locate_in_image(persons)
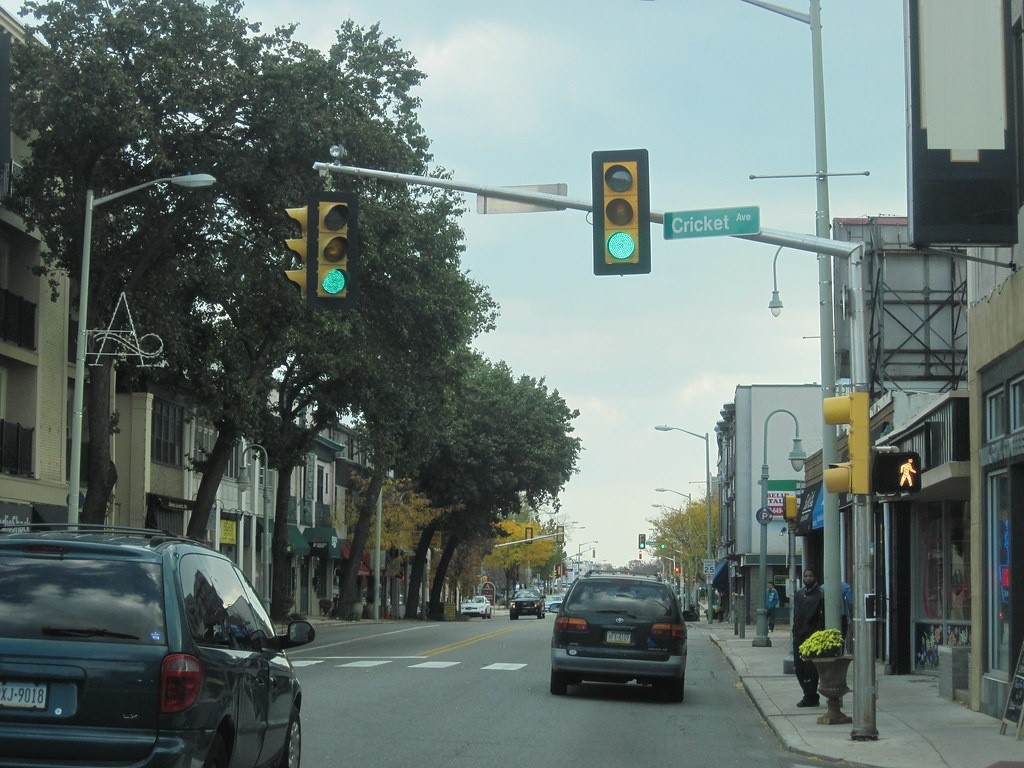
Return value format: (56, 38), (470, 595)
(619, 585), (631, 598)
(765, 581), (780, 633)
(792, 568), (825, 707)
(819, 581), (853, 655)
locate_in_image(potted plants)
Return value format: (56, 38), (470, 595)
(798, 628), (852, 725)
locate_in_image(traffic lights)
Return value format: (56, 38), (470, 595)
(285, 204), (308, 291)
(639, 534), (645, 549)
(316, 200), (353, 299)
(602, 160), (641, 265)
(821, 391), (870, 495)
(876, 450), (921, 494)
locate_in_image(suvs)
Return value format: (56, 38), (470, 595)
(0, 522), (317, 768)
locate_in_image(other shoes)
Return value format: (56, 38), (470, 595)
(797, 695), (819, 707)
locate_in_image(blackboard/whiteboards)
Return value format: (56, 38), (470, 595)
(1002, 641), (1024, 728)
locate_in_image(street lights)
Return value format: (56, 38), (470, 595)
(750, 408), (807, 648)
(235, 443), (271, 620)
(654, 425), (713, 625)
(65, 170), (220, 534)
(655, 487), (691, 613)
(577, 540), (598, 575)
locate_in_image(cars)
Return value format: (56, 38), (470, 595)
(508, 588), (546, 620)
(548, 568), (699, 703)
(460, 595), (492, 620)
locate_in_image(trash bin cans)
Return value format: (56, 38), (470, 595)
(690, 603), (700, 622)
